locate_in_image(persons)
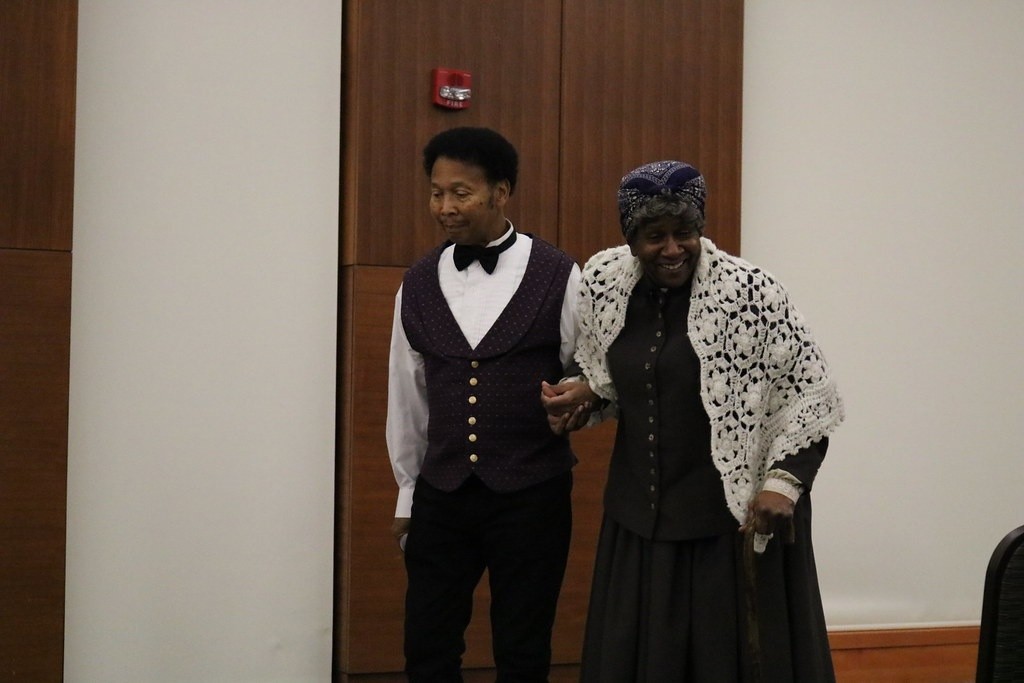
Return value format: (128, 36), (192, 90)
(541, 161), (846, 682)
(385, 127), (592, 683)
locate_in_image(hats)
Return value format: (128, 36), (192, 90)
(618, 160), (708, 233)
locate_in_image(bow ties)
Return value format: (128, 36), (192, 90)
(453, 226), (517, 275)
